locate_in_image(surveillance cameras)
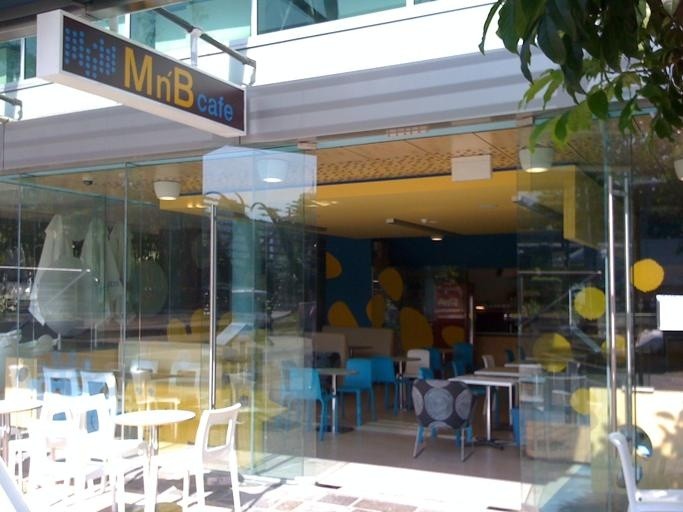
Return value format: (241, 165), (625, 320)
(83, 174), (94, 185)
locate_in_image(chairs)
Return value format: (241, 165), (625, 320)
(0, 388), (243, 511)
(40, 357), (205, 443)
(281, 324), (548, 464)
(167, 305), (238, 338)
(606, 429), (683, 511)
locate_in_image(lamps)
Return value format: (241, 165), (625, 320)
(517, 147), (555, 173)
(153, 179), (184, 202)
(260, 159), (288, 183)
